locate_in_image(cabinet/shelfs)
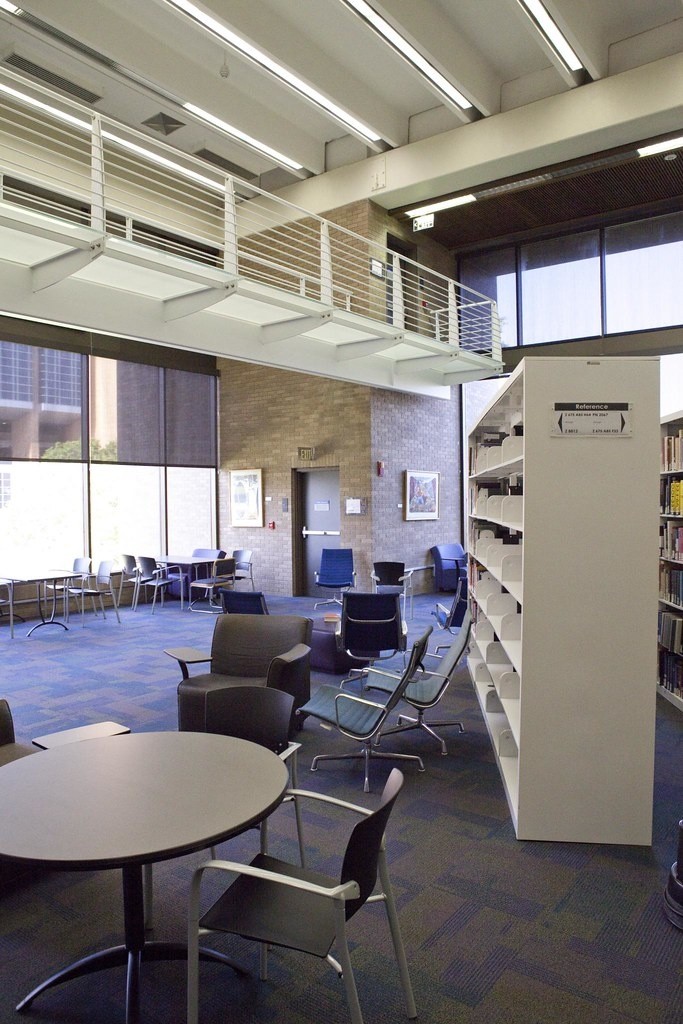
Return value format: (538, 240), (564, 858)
(467, 352), (660, 847)
(659, 409), (683, 713)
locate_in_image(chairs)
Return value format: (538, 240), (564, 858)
(202, 687), (304, 868)
(298, 627), (434, 793)
(0, 699), (44, 767)
(357, 606), (476, 755)
(120, 548), (266, 615)
(431, 577), (468, 663)
(183, 767), (419, 1024)
(369, 560), (414, 620)
(314, 548), (357, 610)
(163, 615), (313, 743)
(44, 558), (119, 626)
(336, 595), (409, 690)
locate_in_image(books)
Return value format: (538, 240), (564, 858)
(659, 430), (683, 697)
(324, 613), (340, 622)
(469, 432), (508, 623)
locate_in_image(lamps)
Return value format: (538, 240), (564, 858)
(219, 51), (230, 79)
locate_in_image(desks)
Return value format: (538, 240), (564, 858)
(155, 558), (236, 605)
(32, 721), (131, 750)
(310, 616), (367, 674)
(0, 732), (290, 1024)
(0, 572), (82, 636)
(163, 645), (212, 680)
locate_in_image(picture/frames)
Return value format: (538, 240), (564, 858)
(228, 469), (266, 528)
(404, 470), (439, 523)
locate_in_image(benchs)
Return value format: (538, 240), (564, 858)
(166, 549), (226, 598)
(431, 541), (469, 593)
